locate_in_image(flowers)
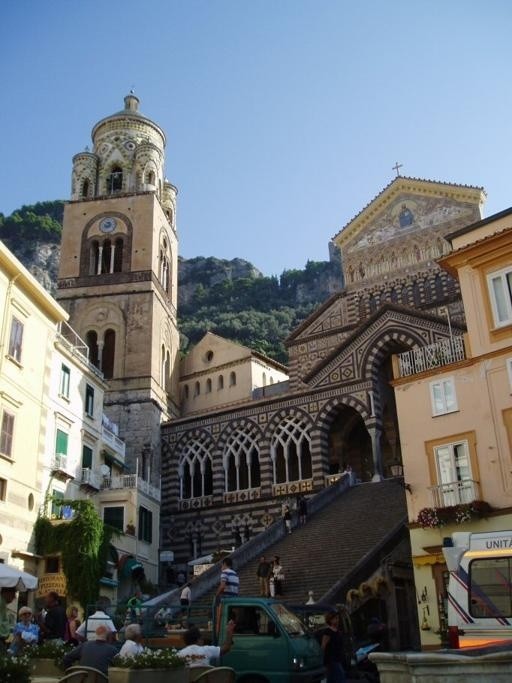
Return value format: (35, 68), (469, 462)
(416, 499), (492, 529)
(1, 638), (76, 682)
(111, 644), (189, 669)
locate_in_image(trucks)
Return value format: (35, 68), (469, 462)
(283, 598), (355, 680)
(364, 528), (512, 683)
(81, 594), (326, 683)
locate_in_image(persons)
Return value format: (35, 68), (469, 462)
(152, 601), (173, 630)
(317, 609), (349, 682)
(255, 555), (271, 597)
(64, 600), (82, 643)
(61, 623), (118, 678)
(8, 605), (43, 665)
(125, 589), (145, 624)
(73, 598), (118, 643)
(179, 580), (193, 617)
(119, 621), (147, 658)
(215, 557), (241, 596)
(36, 590), (67, 644)
(295, 492), (308, 525)
(170, 617), (237, 665)
(284, 503), (293, 534)
(0, 586), (18, 650)
(271, 555), (285, 595)
(267, 573), (276, 597)
